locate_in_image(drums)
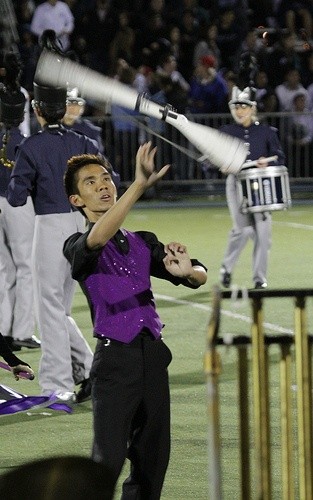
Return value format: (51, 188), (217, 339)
(235, 163), (295, 215)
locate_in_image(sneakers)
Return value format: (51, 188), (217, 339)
(12, 335), (41, 348)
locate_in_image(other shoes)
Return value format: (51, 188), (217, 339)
(253, 282), (264, 289)
(77, 378), (92, 403)
(220, 271), (231, 291)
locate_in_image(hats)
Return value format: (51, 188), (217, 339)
(33, 79), (67, 103)
(228, 86), (257, 108)
(196, 55), (214, 67)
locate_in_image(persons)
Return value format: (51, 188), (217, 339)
(62, 139), (207, 500)
(0, 76), (103, 404)
(12, 0), (313, 200)
(218, 85), (291, 286)
